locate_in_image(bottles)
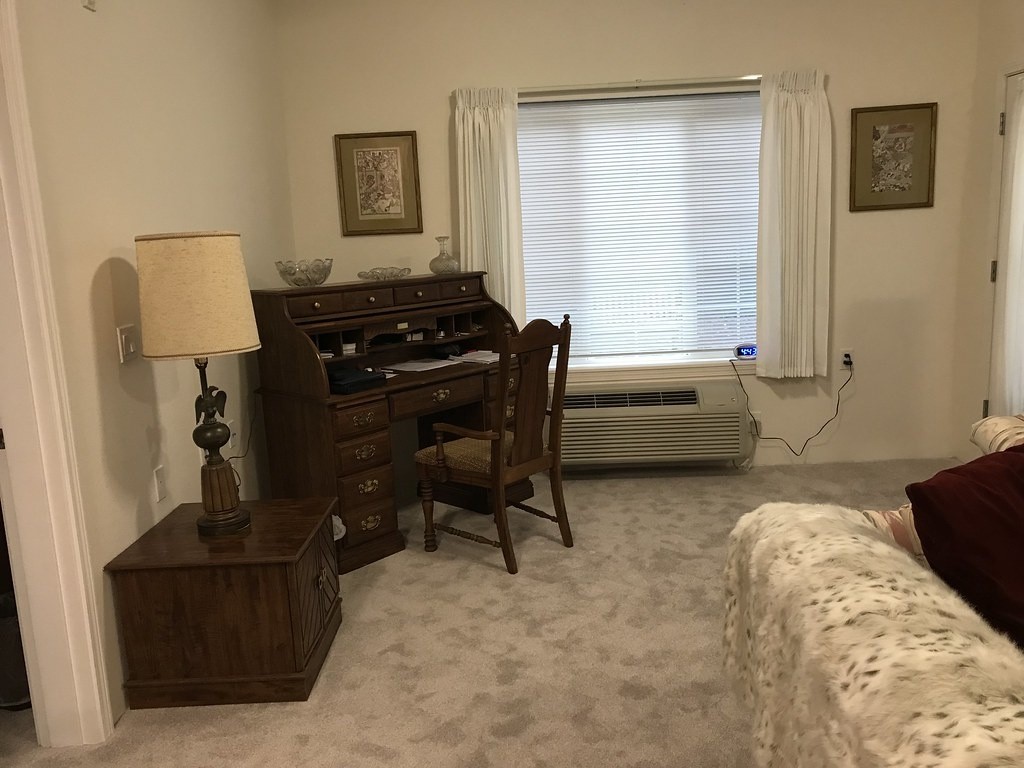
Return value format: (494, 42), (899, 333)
(429, 237), (458, 274)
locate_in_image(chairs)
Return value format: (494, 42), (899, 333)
(411, 313), (574, 574)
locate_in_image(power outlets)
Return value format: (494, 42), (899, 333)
(227, 420), (240, 449)
(747, 412), (763, 433)
(840, 348), (855, 370)
(199, 447), (209, 467)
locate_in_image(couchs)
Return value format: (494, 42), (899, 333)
(721, 415), (1024, 768)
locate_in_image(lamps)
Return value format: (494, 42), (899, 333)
(135, 229), (262, 536)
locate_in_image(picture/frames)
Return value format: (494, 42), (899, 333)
(334, 130), (423, 237)
(849, 102), (939, 211)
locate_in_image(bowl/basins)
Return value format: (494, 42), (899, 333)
(275, 258), (333, 285)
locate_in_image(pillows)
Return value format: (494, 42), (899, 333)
(904, 443), (1024, 654)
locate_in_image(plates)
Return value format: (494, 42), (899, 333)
(357, 267), (411, 281)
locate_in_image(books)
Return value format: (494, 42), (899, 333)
(320, 343), (356, 359)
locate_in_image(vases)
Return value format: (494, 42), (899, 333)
(429, 236), (459, 274)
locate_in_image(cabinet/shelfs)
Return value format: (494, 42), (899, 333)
(103, 493), (344, 711)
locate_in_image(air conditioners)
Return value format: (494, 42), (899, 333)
(549, 379), (749, 465)
